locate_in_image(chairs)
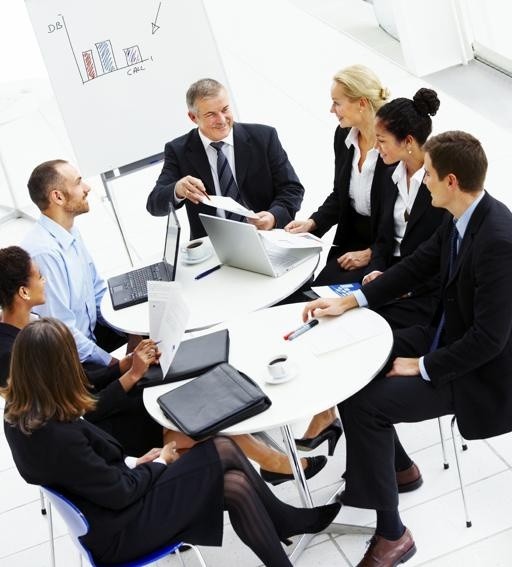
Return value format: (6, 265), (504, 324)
(34, 484), (206, 566)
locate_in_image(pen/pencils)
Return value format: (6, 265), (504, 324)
(127, 340), (162, 358)
(196, 186), (211, 200)
(284, 319), (319, 341)
(195, 263), (224, 280)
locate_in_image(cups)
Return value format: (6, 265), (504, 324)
(180, 240), (206, 261)
(267, 354), (291, 379)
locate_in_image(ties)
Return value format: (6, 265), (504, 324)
(209, 141), (250, 224)
(419, 226), (458, 353)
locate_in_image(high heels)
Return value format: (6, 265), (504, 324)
(275, 502), (342, 546)
(260, 455), (327, 487)
(283, 418), (344, 456)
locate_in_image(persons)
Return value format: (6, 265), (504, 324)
(17, 158), (150, 367)
(304, 127), (511, 567)
(144, 77), (314, 307)
(1, 244), (328, 487)
(282, 63), (391, 287)
(280, 86), (441, 458)
(1, 314), (343, 566)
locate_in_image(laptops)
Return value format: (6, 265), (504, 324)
(198, 211), (322, 278)
(105, 200), (182, 311)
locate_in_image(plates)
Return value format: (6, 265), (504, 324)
(263, 369), (297, 386)
(181, 248), (214, 265)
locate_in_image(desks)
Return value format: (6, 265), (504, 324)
(98, 232), (324, 338)
(138, 298), (395, 563)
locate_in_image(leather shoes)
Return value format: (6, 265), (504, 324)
(355, 524), (417, 567)
(395, 460), (423, 493)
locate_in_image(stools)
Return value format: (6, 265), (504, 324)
(427, 409), (512, 531)
